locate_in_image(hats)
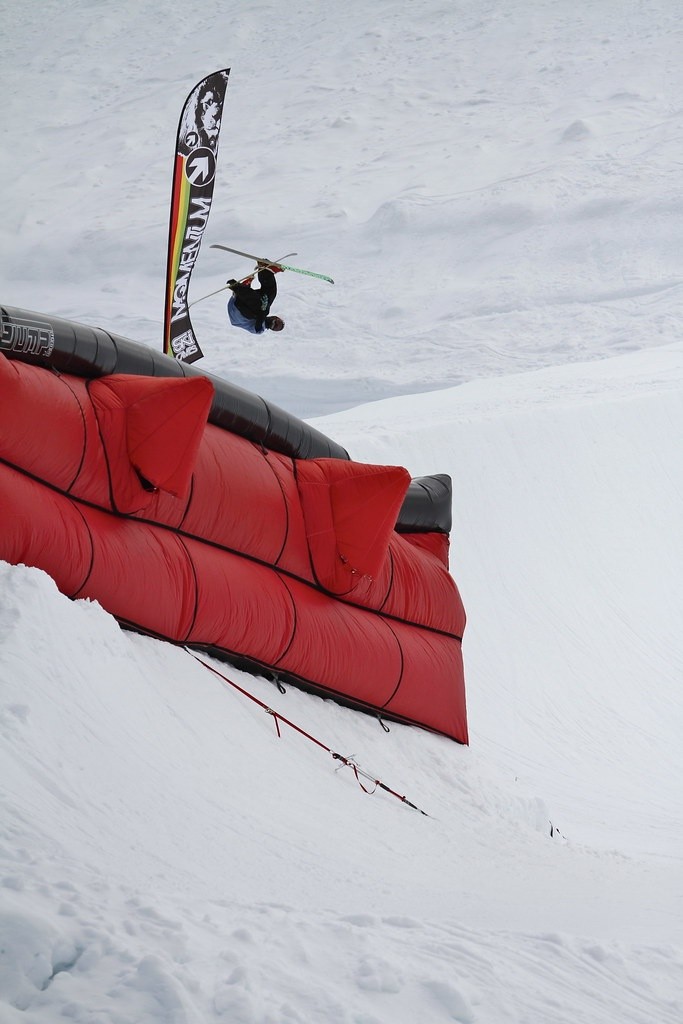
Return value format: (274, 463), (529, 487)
(272, 317), (284, 331)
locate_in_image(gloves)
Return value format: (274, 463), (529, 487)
(226, 279), (237, 285)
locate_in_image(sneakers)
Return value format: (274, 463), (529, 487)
(257, 262), (285, 273)
(242, 276), (254, 286)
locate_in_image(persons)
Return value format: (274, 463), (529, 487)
(226, 259), (284, 334)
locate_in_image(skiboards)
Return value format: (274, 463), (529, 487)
(188, 245), (334, 307)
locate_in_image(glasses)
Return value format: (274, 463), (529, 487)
(272, 318), (276, 330)
